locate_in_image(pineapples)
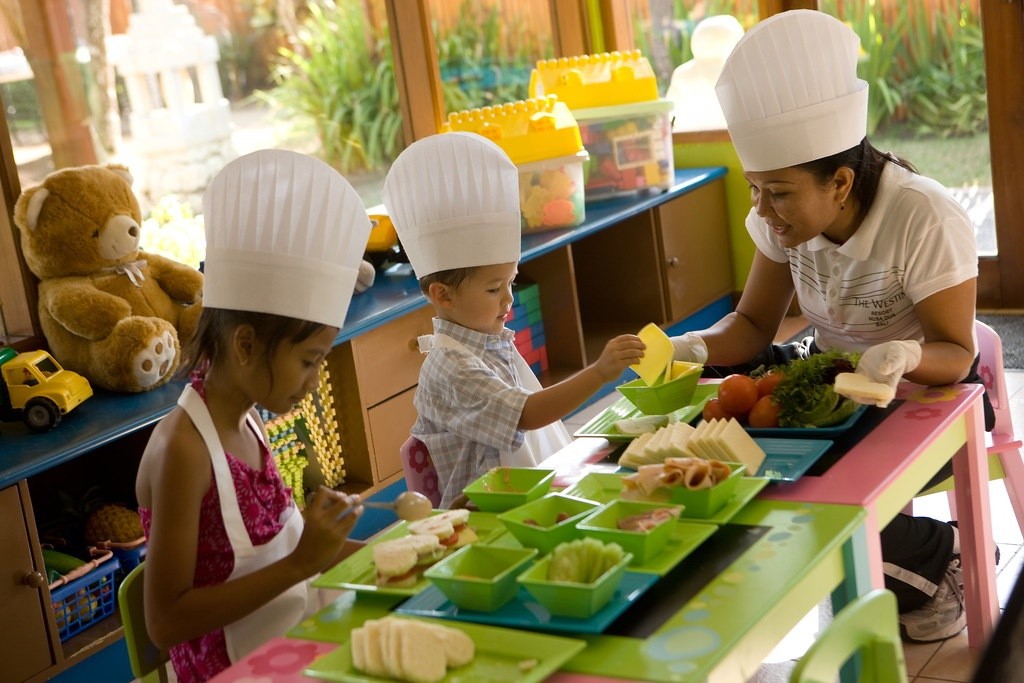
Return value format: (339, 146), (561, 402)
(84, 504), (144, 542)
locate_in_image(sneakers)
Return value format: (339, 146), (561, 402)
(900, 519), (1001, 642)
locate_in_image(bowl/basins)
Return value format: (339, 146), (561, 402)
(497, 491), (604, 556)
(615, 368), (703, 415)
(424, 542), (539, 613)
(668, 460), (747, 518)
(515, 552), (634, 618)
(462, 466), (556, 513)
(576, 499), (685, 565)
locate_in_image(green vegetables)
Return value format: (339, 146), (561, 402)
(748, 349), (867, 429)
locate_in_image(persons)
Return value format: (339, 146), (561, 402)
(135, 150), (373, 683)
(382, 132), (647, 509)
(670, 9), (1001, 642)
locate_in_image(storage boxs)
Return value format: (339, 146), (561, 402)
(43, 521), (147, 579)
(614, 366), (704, 415)
(529, 48), (679, 204)
(647, 461), (746, 519)
(515, 549), (633, 620)
(436, 93), (592, 237)
(496, 491), (604, 557)
(461, 466), (557, 513)
(40, 543), (122, 645)
(422, 542), (539, 613)
(575, 498), (686, 566)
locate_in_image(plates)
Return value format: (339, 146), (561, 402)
(489, 523), (719, 577)
(574, 384), (718, 442)
(393, 571), (659, 633)
(697, 404), (869, 431)
(302, 611), (587, 683)
(561, 472), (769, 525)
(310, 509), (508, 595)
(613, 438), (833, 483)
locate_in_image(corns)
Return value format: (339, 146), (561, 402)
(41, 549), (88, 574)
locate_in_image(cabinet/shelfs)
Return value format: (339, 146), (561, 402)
(0, 161), (732, 682)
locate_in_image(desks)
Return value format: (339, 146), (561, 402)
(202, 636), (658, 683)
(531, 375), (1002, 655)
(283, 485), (871, 683)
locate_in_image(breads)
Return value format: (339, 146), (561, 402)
(833, 373), (893, 400)
(405, 507), (469, 542)
(349, 617), (474, 682)
(372, 534), (439, 577)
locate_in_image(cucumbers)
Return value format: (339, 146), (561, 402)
(549, 536), (625, 585)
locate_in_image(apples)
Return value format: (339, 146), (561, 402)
(54, 576), (109, 629)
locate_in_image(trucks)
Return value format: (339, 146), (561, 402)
(0, 347), (93, 433)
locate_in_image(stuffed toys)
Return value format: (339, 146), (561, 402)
(14, 163), (204, 393)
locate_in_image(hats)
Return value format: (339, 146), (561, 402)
(717, 11), (869, 173)
(203, 148), (374, 330)
(383, 129), (522, 277)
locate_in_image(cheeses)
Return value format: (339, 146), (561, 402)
(618, 416), (767, 478)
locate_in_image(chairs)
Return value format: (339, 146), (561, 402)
(899, 318), (1024, 546)
(788, 587), (909, 683)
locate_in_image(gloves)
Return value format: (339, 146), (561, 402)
(851, 344), (923, 407)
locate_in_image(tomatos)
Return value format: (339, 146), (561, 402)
(701, 372), (785, 428)
(439, 524), (464, 544)
(388, 566), (418, 583)
